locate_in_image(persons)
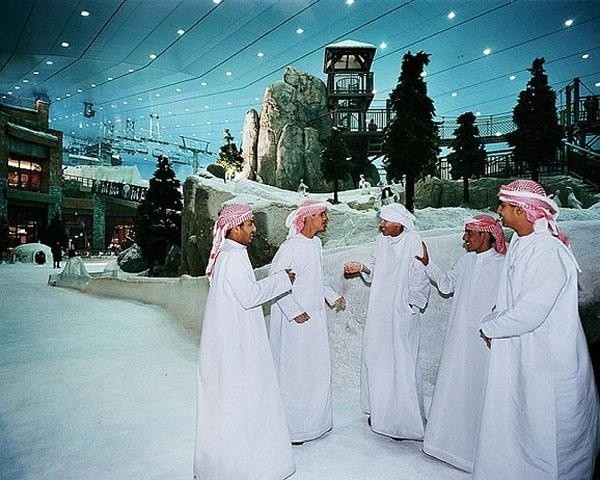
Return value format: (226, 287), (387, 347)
(477, 181), (598, 479)
(269, 199), (346, 445)
(368, 119), (377, 133)
(51, 241), (62, 268)
(343, 203), (431, 442)
(414, 214), (506, 473)
(193, 203), (296, 480)
